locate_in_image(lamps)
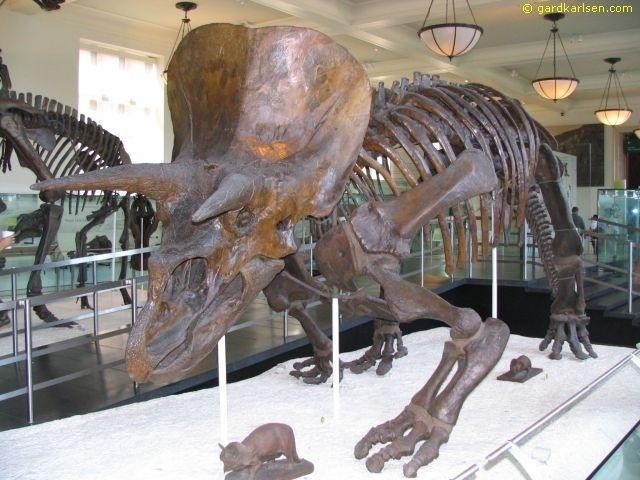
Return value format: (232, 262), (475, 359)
(594, 58), (633, 128)
(532, 12), (580, 103)
(160, 2), (197, 87)
(417, 0), (484, 62)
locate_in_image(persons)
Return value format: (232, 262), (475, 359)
(571, 205), (587, 240)
(589, 214), (605, 255)
(0, 234), (17, 252)
